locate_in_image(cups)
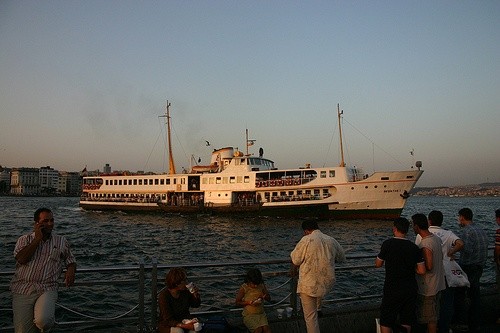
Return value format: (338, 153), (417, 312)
(187, 282), (195, 294)
(277, 309), (285, 318)
(287, 307), (293, 317)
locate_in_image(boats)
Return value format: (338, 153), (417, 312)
(78, 99), (425, 220)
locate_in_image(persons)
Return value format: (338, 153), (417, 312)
(493, 209), (500, 324)
(235, 268), (272, 333)
(155, 191), (204, 206)
(289, 219), (345, 333)
(159, 269), (201, 333)
(375, 217), (426, 333)
(9, 208), (77, 333)
(256, 175), (300, 188)
(413, 210), (464, 333)
(237, 193), (261, 206)
(452, 207), (489, 331)
(410, 212), (444, 333)
